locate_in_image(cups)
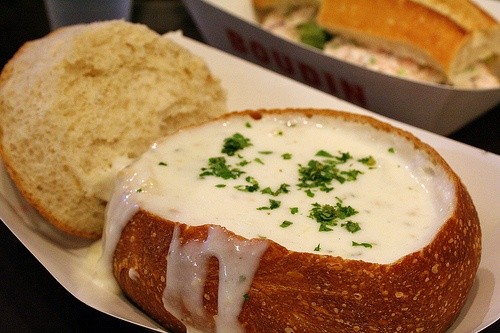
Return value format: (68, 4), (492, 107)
(44, 0), (132, 33)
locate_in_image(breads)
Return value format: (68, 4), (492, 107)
(0, 19), (228, 240)
(250, 0), (500, 91)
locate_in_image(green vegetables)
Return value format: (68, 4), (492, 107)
(157, 122), (395, 252)
